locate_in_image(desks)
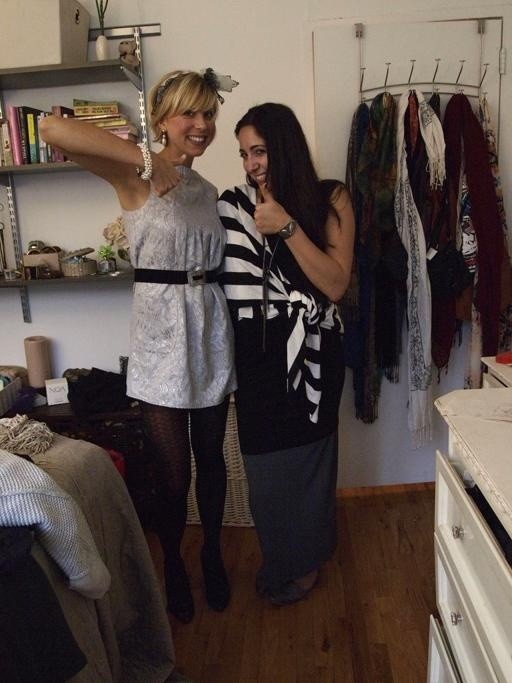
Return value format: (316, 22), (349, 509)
(9, 387), (161, 528)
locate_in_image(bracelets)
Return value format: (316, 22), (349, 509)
(273, 214), (300, 242)
(135, 138), (153, 181)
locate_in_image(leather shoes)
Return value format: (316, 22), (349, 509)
(269, 568), (319, 605)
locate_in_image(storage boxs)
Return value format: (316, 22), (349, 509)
(0, 0), (92, 70)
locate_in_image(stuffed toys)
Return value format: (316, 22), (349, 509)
(114, 39), (139, 65)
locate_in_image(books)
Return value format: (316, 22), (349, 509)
(0, 100), (139, 167)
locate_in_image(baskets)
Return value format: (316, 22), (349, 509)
(62, 259), (97, 276)
(186, 399), (257, 528)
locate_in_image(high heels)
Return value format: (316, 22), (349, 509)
(197, 543), (230, 615)
(166, 564), (192, 622)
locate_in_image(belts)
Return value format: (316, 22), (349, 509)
(131, 266), (219, 286)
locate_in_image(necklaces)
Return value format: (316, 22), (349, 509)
(261, 234), (279, 354)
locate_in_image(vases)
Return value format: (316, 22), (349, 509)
(95, 258), (117, 274)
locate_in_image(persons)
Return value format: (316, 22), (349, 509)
(35, 69), (238, 623)
(217, 100), (357, 604)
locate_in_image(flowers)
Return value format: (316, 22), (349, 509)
(99, 215), (130, 260)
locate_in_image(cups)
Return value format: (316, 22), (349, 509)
(23, 335), (51, 389)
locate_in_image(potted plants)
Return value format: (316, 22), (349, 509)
(96, 0), (110, 60)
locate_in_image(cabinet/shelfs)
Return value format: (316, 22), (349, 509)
(0, 57), (149, 287)
(427, 385), (512, 682)
(474, 354), (512, 387)
